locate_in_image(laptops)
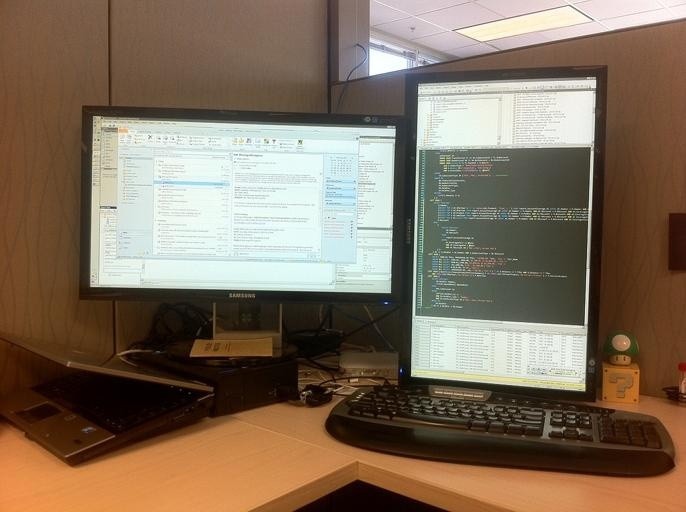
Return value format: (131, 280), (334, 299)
(0, 333), (216, 466)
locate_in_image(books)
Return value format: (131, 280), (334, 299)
(189, 339), (275, 358)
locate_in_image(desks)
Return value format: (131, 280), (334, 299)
(0, 370), (686, 512)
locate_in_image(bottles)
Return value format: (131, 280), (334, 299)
(678, 362), (686, 406)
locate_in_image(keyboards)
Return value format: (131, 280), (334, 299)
(325, 385), (676, 478)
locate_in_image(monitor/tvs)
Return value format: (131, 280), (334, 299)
(402, 64), (607, 401)
(78, 105), (404, 368)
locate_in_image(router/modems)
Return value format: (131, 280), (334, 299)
(212, 299), (282, 349)
(338, 350), (398, 380)
